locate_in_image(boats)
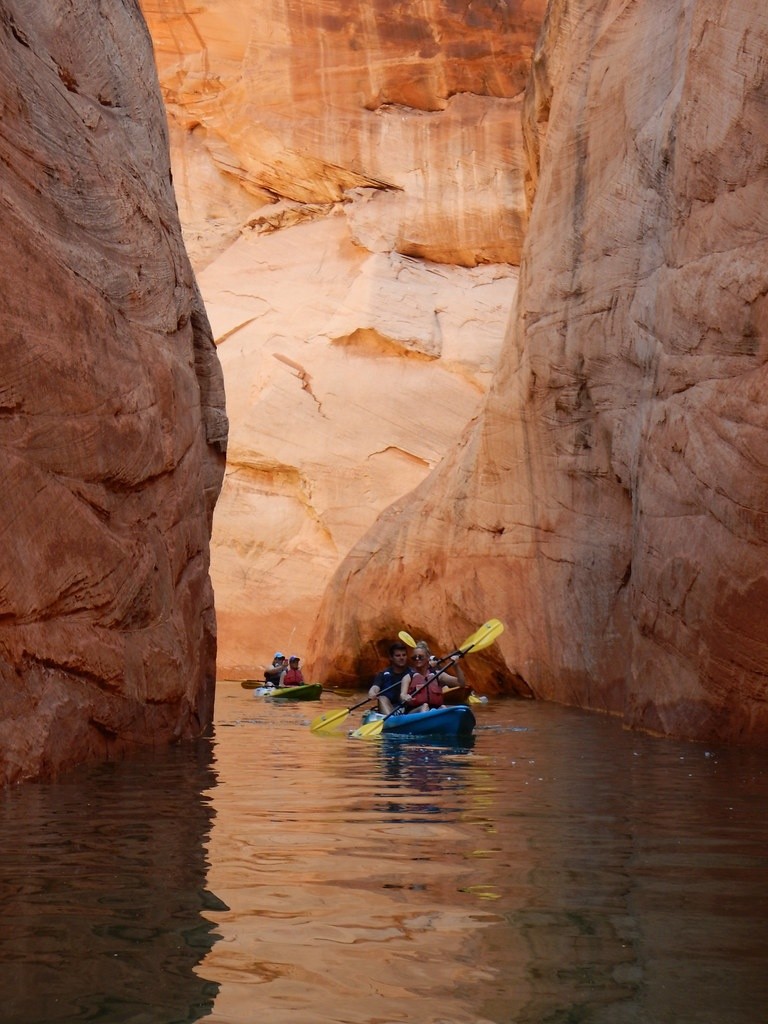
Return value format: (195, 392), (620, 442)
(361, 703), (476, 738)
(253, 682), (323, 700)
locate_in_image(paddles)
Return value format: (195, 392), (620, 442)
(397, 630), (482, 704)
(310, 631), (478, 733)
(223, 677), (324, 688)
(350, 617), (506, 737)
(240, 680), (353, 697)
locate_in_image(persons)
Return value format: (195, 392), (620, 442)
(368, 645), (440, 716)
(400, 641), (465, 713)
(264, 653), (289, 689)
(277, 656), (304, 687)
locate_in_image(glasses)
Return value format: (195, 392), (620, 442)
(411, 655), (425, 661)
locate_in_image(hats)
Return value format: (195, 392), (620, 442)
(290, 656), (300, 661)
(275, 653), (284, 658)
